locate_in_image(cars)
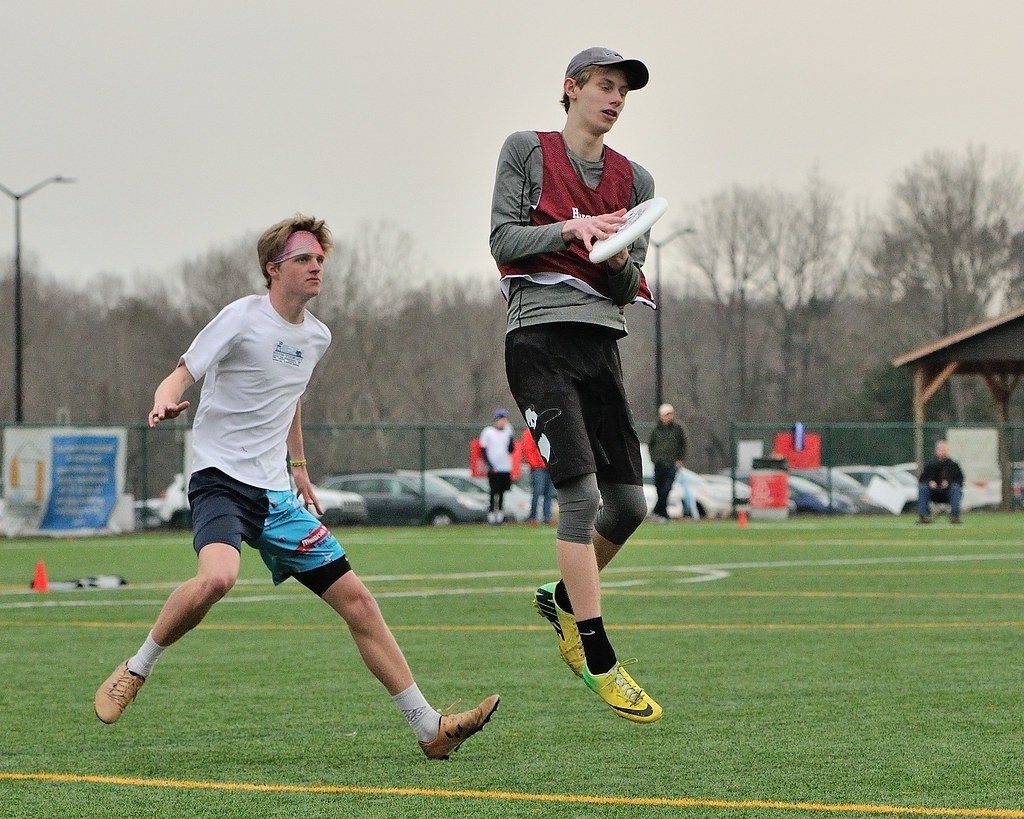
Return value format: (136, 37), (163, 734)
(318, 467), (492, 528)
(637, 445), (794, 521)
(803, 464), (939, 517)
(430, 469), (543, 523)
(720, 469), (856, 515)
(158, 468), (367, 528)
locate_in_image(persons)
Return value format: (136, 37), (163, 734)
(522, 425), (558, 526)
(916, 439), (965, 524)
(95, 216), (502, 759)
(490, 47), (663, 722)
(648, 404), (687, 523)
(159, 473), (186, 525)
(480, 409), (513, 524)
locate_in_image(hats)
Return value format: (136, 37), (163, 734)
(659, 404), (674, 416)
(565, 47), (649, 91)
(493, 410), (510, 420)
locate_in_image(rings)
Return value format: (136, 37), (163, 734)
(152, 414), (158, 418)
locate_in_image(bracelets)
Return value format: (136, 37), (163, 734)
(289, 460), (306, 466)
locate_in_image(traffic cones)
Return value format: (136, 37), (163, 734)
(33, 561), (50, 591)
(736, 511), (749, 526)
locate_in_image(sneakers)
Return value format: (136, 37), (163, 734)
(533, 580), (586, 679)
(94, 657), (145, 724)
(582, 657), (663, 723)
(417, 694), (501, 761)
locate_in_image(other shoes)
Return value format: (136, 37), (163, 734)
(528, 519), (537, 527)
(488, 509), (504, 524)
(545, 519), (557, 526)
(648, 510), (667, 525)
(921, 515), (931, 523)
(950, 516), (959, 525)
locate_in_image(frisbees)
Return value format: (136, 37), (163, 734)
(588, 194), (669, 265)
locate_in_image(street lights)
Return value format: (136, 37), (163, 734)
(0, 170), (85, 422)
(644, 227), (702, 429)
